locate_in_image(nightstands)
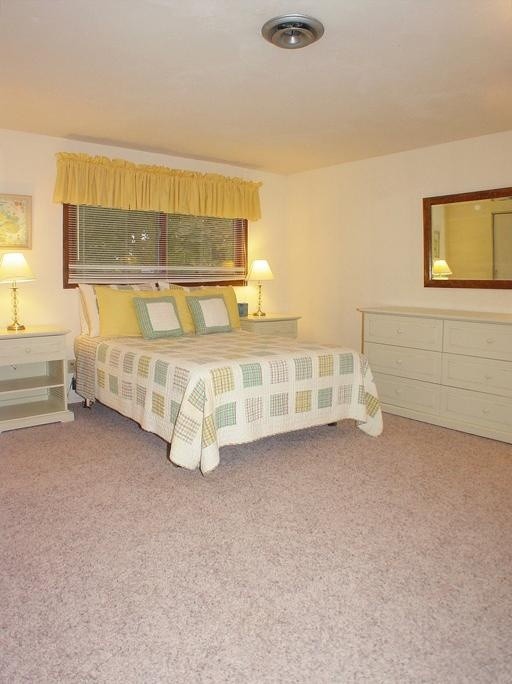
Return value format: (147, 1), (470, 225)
(239, 313), (303, 340)
(0, 325), (77, 433)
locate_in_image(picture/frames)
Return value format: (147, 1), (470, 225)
(430, 228), (441, 262)
(0, 191), (35, 250)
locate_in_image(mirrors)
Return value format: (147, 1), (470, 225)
(421, 186), (512, 293)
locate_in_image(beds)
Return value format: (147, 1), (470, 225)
(70, 281), (384, 476)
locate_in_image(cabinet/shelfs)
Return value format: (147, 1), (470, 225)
(356, 302), (512, 450)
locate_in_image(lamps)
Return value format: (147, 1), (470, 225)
(0, 249), (42, 332)
(245, 257), (273, 317)
(432, 257), (453, 281)
(262, 12), (323, 52)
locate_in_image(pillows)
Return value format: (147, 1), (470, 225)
(132, 294), (183, 340)
(170, 285), (241, 334)
(94, 284), (184, 340)
(187, 292), (234, 336)
(156, 283), (215, 293)
(76, 281), (158, 339)
(79, 307), (89, 336)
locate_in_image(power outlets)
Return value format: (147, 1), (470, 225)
(66, 358), (75, 375)
